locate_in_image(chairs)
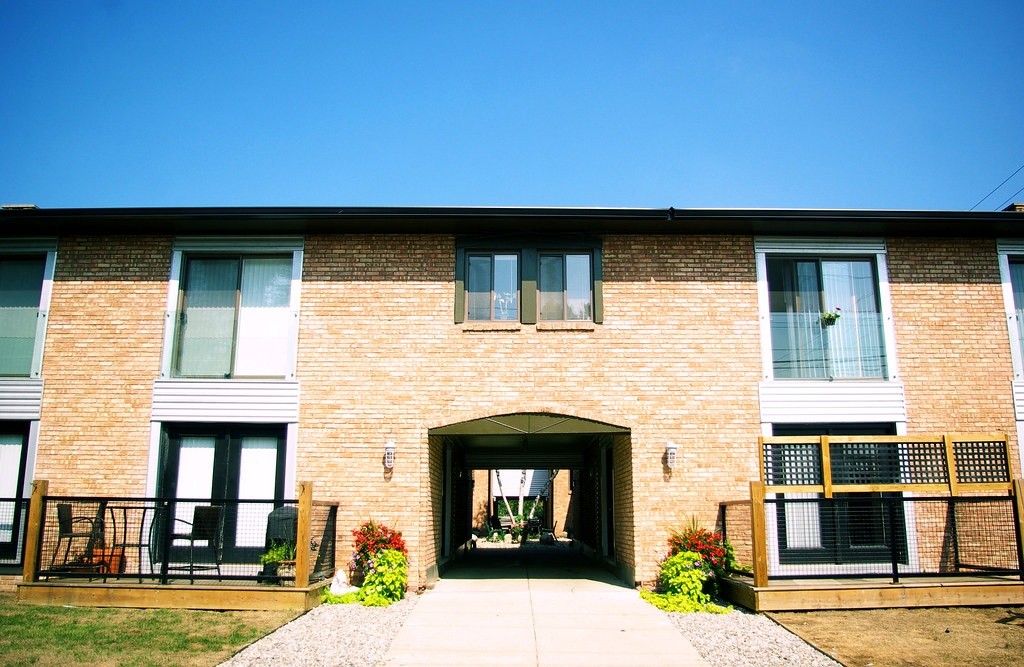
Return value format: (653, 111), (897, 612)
(485, 521), (504, 541)
(540, 521), (558, 542)
(46, 504), (106, 583)
(162, 506), (222, 585)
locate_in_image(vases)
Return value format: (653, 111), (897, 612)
(825, 319), (836, 325)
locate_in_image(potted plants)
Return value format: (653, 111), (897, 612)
(259, 522), (297, 586)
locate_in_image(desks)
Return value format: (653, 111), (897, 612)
(107, 506), (161, 584)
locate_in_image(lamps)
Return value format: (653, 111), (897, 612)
(384, 439), (397, 469)
(665, 443), (679, 478)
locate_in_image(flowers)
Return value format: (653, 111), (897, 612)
(818, 307), (841, 320)
